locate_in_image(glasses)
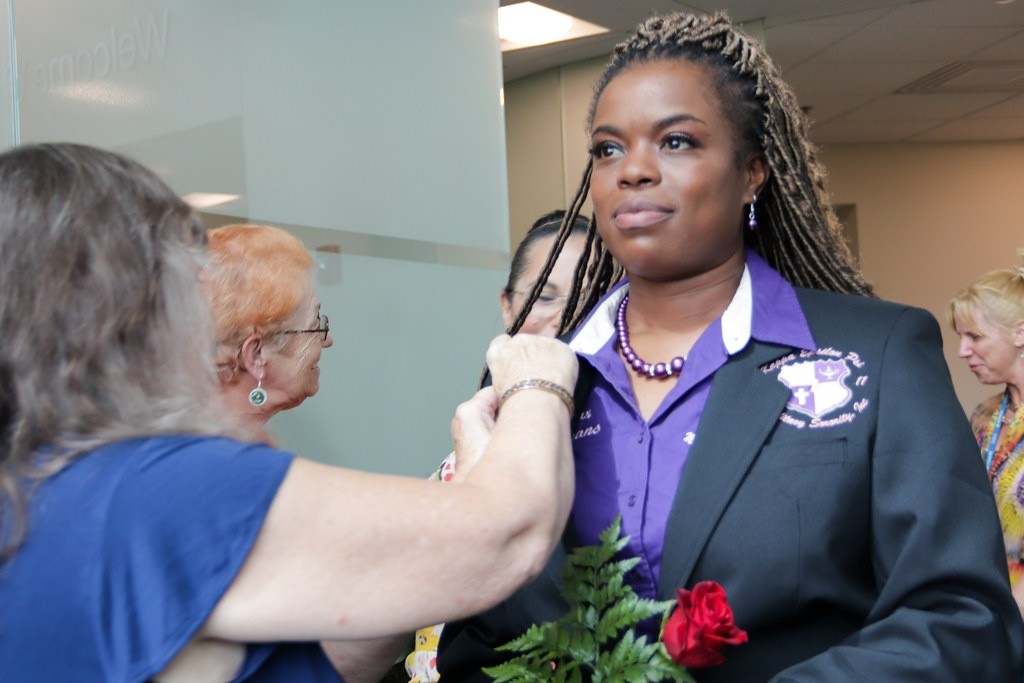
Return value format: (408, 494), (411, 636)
(514, 289), (585, 313)
(263, 315), (329, 341)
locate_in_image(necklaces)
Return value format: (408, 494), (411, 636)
(618, 297), (685, 377)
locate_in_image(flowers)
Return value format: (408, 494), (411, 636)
(481, 511), (749, 683)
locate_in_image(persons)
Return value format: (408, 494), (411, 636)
(404, 208), (626, 683)
(436, 11), (1024, 683)
(198, 224), (333, 447)
(0, 142), (579, 683)
(947, 267), (1024, 623)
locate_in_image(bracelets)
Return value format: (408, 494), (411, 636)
(498, 379), (575, 415)
